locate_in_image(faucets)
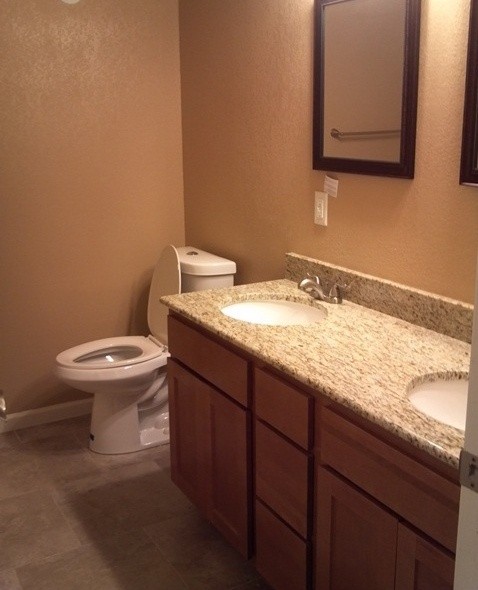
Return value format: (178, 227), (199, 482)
(298, 279), (326, 300)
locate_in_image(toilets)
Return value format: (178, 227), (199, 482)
(53, 244), (236, 456)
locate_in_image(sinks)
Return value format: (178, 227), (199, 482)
(216, 291), (330, 326)
(402, 369), (469, 434)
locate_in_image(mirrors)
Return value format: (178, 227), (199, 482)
(311, 1), (422, 180)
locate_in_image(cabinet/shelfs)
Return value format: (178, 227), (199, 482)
(165, 310), (254, 559)
(251, 365), (314, 589)
(315, 404), (459, 590)
